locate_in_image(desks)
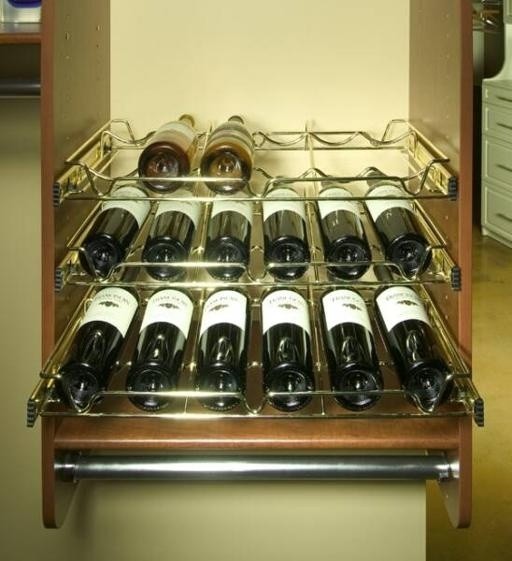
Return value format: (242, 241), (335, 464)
(0, 22), (40, 99)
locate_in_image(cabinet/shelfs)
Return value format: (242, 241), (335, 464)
(26, 0), (483, 530)
(482, 79), (512, 249)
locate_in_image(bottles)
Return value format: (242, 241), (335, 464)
(53, 287), (142, 412)
(123, 284), (195, 413)
(199, 188), (255, 281)
(316, 285), (385, 412)
(363, 171), (434, 277)
(314, 181), (374, 281)
(192, 283), (251, 412)
(136, 113), (199, 195)
(77, 179), (152, 280)
(140, 187), (203, 281)
(199, 115), (257, 196)
(255, 283), (315, 414)
(372, 284), (457, 411)
(260, 183), (312, 281)
